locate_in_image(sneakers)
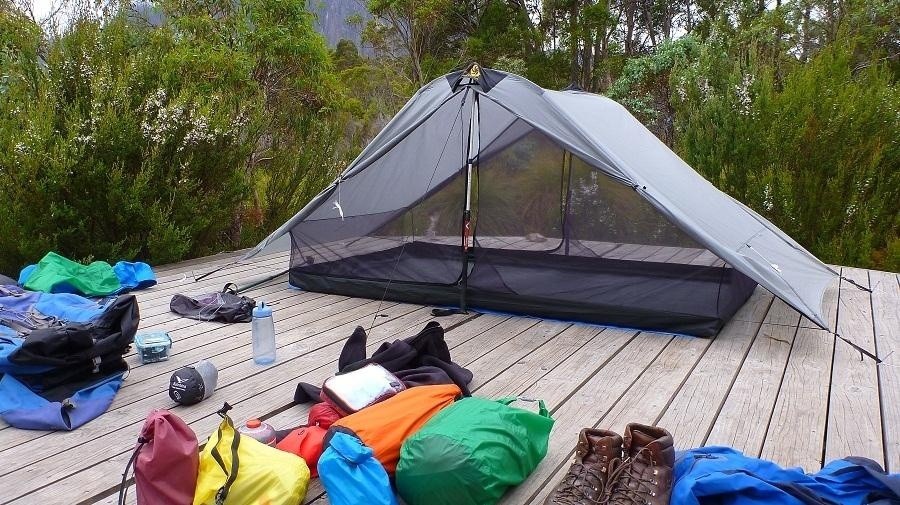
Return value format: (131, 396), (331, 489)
(607, 422), (677, 505)
(543, 427), (622, 505)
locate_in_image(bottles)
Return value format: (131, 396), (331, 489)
(251, 299), (276, 366)
(238, 418), (276, 449)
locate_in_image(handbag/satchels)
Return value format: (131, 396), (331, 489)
(192, 397), (312, 505)
(168, 281), (256, 324)
(392, 394), (556, 504)
(129, 406), (199, 505)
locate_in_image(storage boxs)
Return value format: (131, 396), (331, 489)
(134, 329), (170, 364)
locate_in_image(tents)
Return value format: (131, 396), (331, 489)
(193, 61), (882, 364)
